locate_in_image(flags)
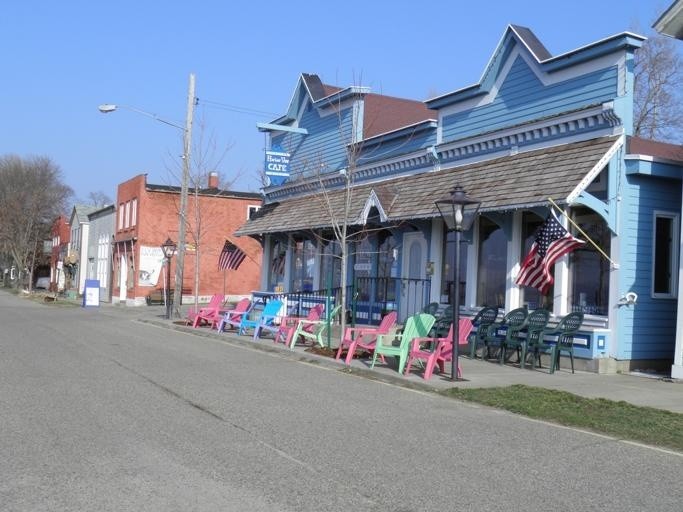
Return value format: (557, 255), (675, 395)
(512, 208), (590, 295)
(215, 239), (246, 271)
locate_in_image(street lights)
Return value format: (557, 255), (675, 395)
(432, 181), (483, 386)
(95, 101), (193, 320)
(159, 235), (178, 321)
